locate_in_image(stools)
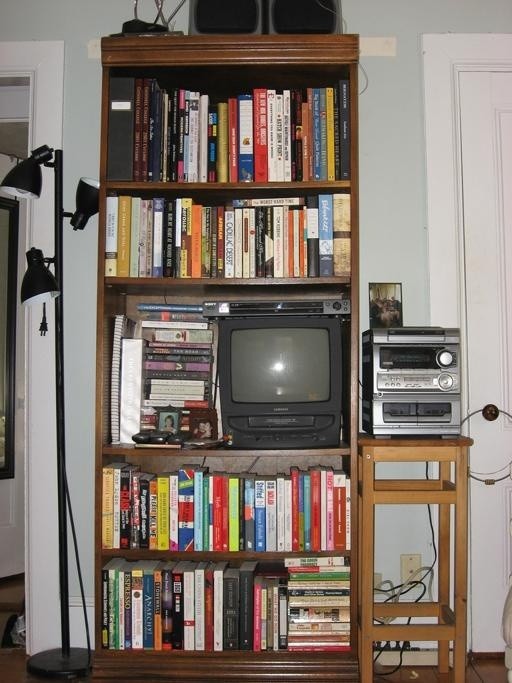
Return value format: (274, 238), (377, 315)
(357, 435), (471, 683)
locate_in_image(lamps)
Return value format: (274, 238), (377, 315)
(1, 141), (99, 679)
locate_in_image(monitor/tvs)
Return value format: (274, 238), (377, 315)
(218, 319), (343, 450)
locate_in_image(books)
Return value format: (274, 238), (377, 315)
(102, 462), (352, 653)
(111, 303), (214, 448)
(104, 193), (350, 278)
(107, 77), (351, 184)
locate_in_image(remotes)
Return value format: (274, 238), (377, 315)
(168, 430), (192, 445)
(150, 431), (173, 444)
(132, 430), (151, 444)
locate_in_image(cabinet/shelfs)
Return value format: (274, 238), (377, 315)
(93, 32), (363, 682)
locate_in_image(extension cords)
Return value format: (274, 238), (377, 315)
(373, 648), (469, 666)
